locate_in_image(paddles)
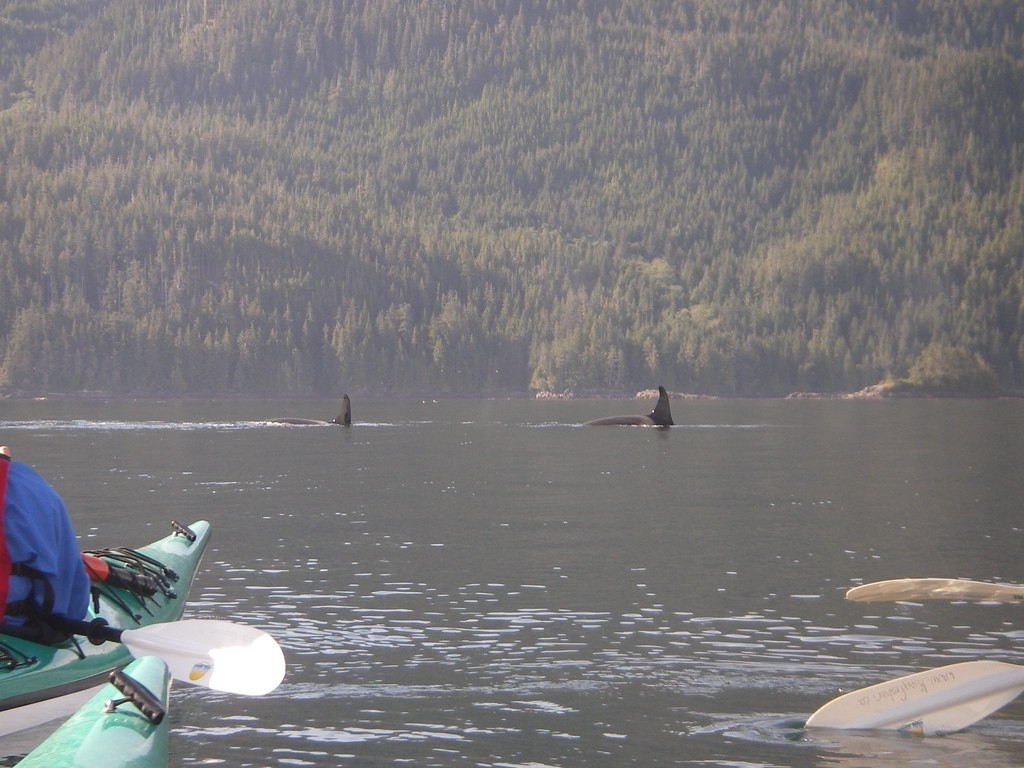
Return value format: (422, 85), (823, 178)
(33, 610), (289, 699)
(845, 576), (1024, 602)
(803, 658), (1024, 738)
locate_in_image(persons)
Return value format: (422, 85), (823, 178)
(0, 446), (91, 645)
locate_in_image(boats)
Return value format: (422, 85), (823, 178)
(0, 520), (212, 739)
(10, 654), (172, 767)
